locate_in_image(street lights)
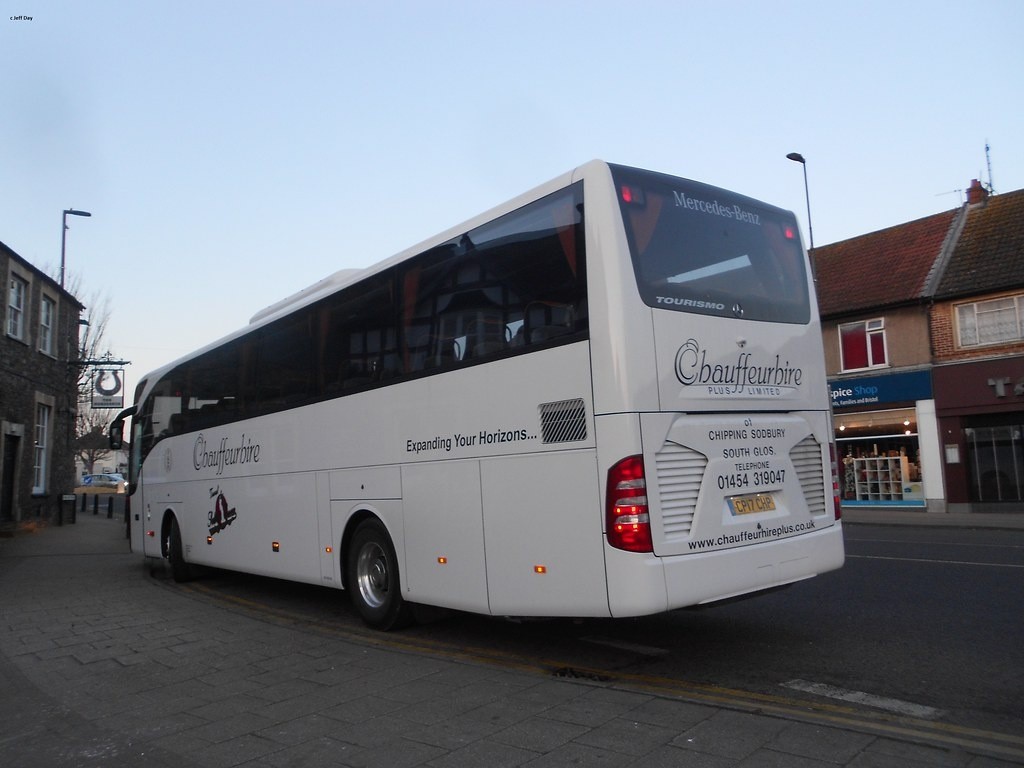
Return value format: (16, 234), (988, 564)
(787, 151), (817, 302)
(57, 208), (93, 525)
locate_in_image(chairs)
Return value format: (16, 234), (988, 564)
(157, 296), (583, 439)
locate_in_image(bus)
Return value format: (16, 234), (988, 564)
(107, 159), (851, 634)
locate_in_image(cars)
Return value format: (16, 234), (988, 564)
(82, 474), (129, 489)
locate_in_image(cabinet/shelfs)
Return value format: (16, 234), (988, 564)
(854, 456), (910, 501)
